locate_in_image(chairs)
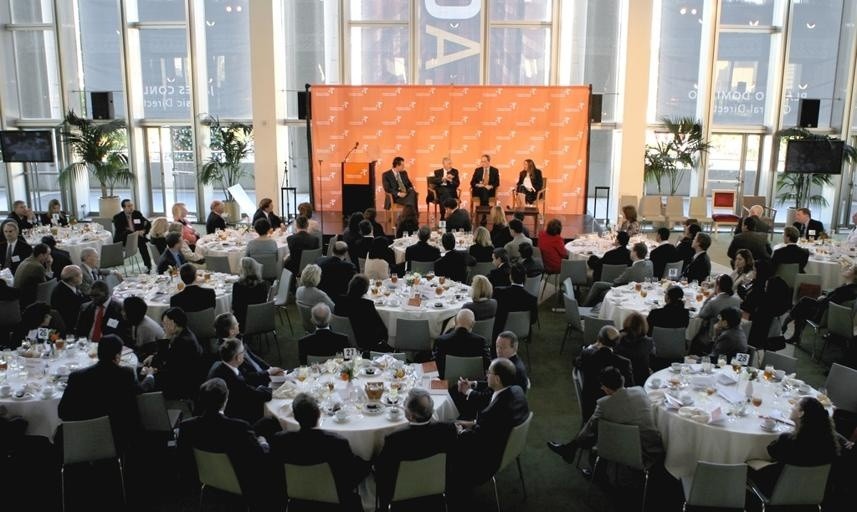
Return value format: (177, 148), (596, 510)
(297, 302), (316, 335)
(503, 311), (531, 375)
(468, 261), (497, 284)
(284, 462), (341, 512)
(512, 177), (548, 219)
(474, 412), (533, 512)
(793, 275), (822, 303)
(413, 260), (434, 275)
(93, 217), (113, 233)
(471, 318), (495, 364)
(584, 282), (615, 307)
(312, 229), (322, 247)
(663, 261), (684, 281)
(642, 197), (665, 232)
(581, 316), (615, 345)
(828, 301), (856, 338)
(806, 309), (828, 359)
(589, 420), (666, 512)
(0, 298), (20, 325)
(743, 195), (777, 240)
(298, 249), (322, 276)
(383, 191), (404, 227)
(683, 462), (747, 512)
(146, 241), (161, 265)
(741, 265), (768, 320)
(204, 255), (239, 277)
(124, 232), (141, 274)
(653, 325), (690, 360)
(240, 301), (281, 366)
(427, 178), (460, 217)
(272, 268), (292, 334)
(278, 248), (286, 279)
(98, 241), (127, 280)
(525, 274), (543, 331)
(760, 351), (797, 375)
(559, 259), (588, 295)
(560, 277), (597, 354)
(747, 344), (759, 368)
(563, 293), (596, 332)
(748, 463), (831, 512)
(469, 187), (497, 218)
(521, 247), (541, 265)
(687, 196), (713, 235)
(104, 273), (118, 294)
(330, 314), (365, 354)
(741, 319), (753, 340)
(386, 452), (449, 512)
(665, 196), (688, 232)
(824, 362), (857, 411)
(445, 354), (482, 390)
(185, 307), (217, 356)
(620, 195), (643, 229)
(775, 264), (799, 287)
(395, 318), (432, 362)
(322, 233), (339, 259)
(602, 265), (628, 282)
(711, 190), (742, 237)
(572, 367), (587, 467)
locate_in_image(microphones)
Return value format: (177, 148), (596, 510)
(343, 141), (359, 163)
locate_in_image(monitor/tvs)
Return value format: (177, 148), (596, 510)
(0, 129), (55, 163)
(784, 137), (846, 176)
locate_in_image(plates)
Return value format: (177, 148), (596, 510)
(12, 390), (32, 400)
(383, 393), (402, 406)
(362, 401), (384, 415)
(668, 362), (685, 373)
(760, 417), (778, 432)
(360, 366), (382, 378)
(332, 410), (351, 425)
(646, 378), (661, 390)
(385, 407), (405, 421)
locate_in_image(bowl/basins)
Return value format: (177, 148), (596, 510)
(763, 420), (775, 429)
(773, 369), (786, 378)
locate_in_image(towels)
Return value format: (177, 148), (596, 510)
(647, 390), (670, 402)
(783, 373), (804, 386)
(678, 406), (707, 422)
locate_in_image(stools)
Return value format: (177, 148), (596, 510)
(137, 391), (182, 441)
(37, 277), (56, 302)
(57, 416), (129, 512)
(192, 446), (251, 512)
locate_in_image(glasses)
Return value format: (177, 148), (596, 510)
(486, 370), (497, 376)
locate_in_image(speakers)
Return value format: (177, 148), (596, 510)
(90, 92), (115, 120)
(591, 94), (603, 124)
(797, 98), (820, 129)
(296, 90), (311, 120)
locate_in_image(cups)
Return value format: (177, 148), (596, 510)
(0, 360), (8, 374)
(384, 288), (391, 300)
(66, 335), (75, 350)
(337, 414), (348, 421)
(704, 290), (709, 300)
(78, 336), (88, 351)
(391, 273), (398, 286)
(817, 386), (828, 406)
(732, 361), (741, 373)
(763, 365), (774, 385)
(204, 274), (211, 286)
(435, 286), (444, 299)
(439, 277), (444, 286)
(178, 282), (184, 291)
(444, 284), (449, 294)
(652, 277), (658, 289)
(427, 272), (432, 284)
(718, 353), (726, 373)
(641, 289), (648, 303)
(371, 287), (378, 298)
(751, 395), (762, 417)
(297, 364), (308, 386)
(701, 356), (709, 376)
(390, 410), (402, 419)
(375, 278), (382, 289)
(671, 363), (682, 370)
(636, 284), (641, 297)
(696, 294), (703, 307)
(55, 339), (64, 358)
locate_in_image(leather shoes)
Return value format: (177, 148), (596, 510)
(582, 467), (595, 481)
(546, 441), (574, 464)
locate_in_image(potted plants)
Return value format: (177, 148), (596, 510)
(197, 111), (256, 225)
(774, 128), (857, 228)
(56, 106), (136, 217)
(644, 114), (715, 229)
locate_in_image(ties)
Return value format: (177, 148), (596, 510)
(484, 169), (489, 186)
(396, 172), (406, 192)
(5, 244), (13, 270)
(92, 306), (104, 342)
(800, 224), (806, 237)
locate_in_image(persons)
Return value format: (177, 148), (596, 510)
(470, 154), (500, 225)
(433, 157), (460, 202)
(495, 267), (539, 331)
(168, 221), (204, 262)
(296, 302), (352, 366)
(545, 365), (665, 479)
(211, 311), (286, 387)
(295, 263), (335, 314)
(244, 217), (280, 280)
(449, 331), (528, 413)
(381, 156), (417, 204)
(0, 221), (33, 300)
(9, 301), (66, 350)
(317, 240), (354, 303)
(172, 203), (200, 244)
(49, 264), (89, 323)
(272, 392), (370, 489)
(284, 216), (320, 273)
(113, 198), (152, 270)
(206, 338), (274, 425)
(334, 273), (389, 351)
(461, 274), (498, 321)
(7, 200), (38, 234)
(76, 246), (124, 288)
(169, 263), (217, 312)
(291, 202), (322, 235)
(514, 158), (544, 212)
(342, 199), (543, 285)
(617, 311), (661, 385)
(13, 243), (52, 296)
(206, 199), (227, 235)
(231, 256), (268, 332)
(585, 203), (857, 335)
(157, 231), (188, 274)
(453, 356), (530, 475)
(747, 396), (839, 497)
(431, 308), (492, 380)
(252, 198), (285, 231)
(41, 199), (68, 227)
(149, 216), (170, 254)
(41, 235), (73, 279)
(688, 306), (748, 367)
(75, 281), (131, 342)
(537, 218), (570, 274)
(120, 296), (165, 352)
(175, 377), (282, 485)
(370, 388), (460, 481)
(52, 333), (141, 453)
(571, 324), (636, 421)
(137, 306), (204, 398)
